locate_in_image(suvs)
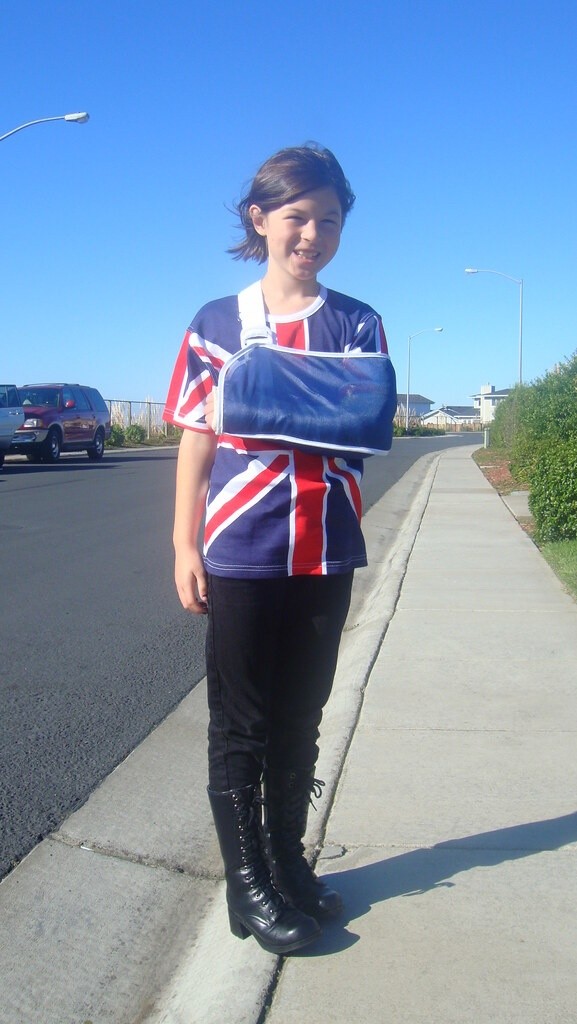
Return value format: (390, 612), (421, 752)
(6, 382), (112, 464)
(0, 384), (26, 468)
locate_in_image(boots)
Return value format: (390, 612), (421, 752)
(205, 782), (325, 957)
(261, 765), (346, 924)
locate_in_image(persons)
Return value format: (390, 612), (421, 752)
(163, 143), (399, 955)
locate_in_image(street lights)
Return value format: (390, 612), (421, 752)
(465, 267), (523, 392)
(405, 328), (444, 431)
